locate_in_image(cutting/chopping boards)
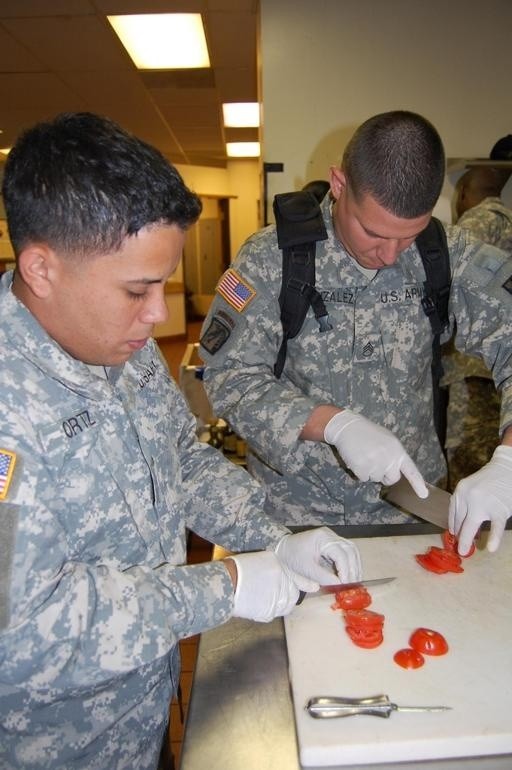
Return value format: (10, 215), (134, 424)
(269, 531), (511, 769)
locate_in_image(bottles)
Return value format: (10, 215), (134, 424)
(206, 421), (246, 460)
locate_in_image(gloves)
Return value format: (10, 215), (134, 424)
(221, 550), (323, 625)
(323, 407), (433, 502)
(447, 444), (511, 557)
(274, 524), (363, 595)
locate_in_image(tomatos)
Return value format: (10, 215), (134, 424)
(331, 585), (388, 649)
(413, 530), (478, 573)
(394, 627), (449, 671)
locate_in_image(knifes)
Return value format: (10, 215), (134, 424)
(293, 575), (397, 608)
(377, 466), (472, 537)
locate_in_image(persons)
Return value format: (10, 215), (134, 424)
(199, 111), (511, 557)
(1, 110), (364, 770)
(445, 165), (511, 494)
